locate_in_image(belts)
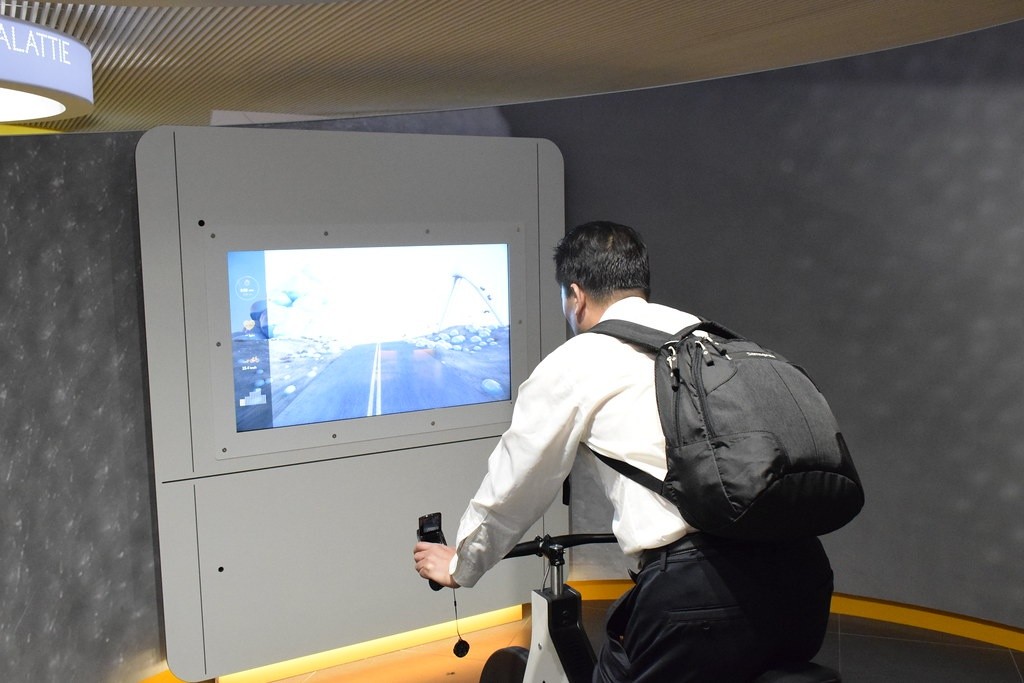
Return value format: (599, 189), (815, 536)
(643, 532), (713, 566)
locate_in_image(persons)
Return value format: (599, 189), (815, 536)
(414, 222), (833, 683)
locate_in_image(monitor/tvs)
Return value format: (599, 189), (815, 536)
(224, 242), (512, 434)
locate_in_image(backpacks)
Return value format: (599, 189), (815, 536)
(581, 315), (865, 547)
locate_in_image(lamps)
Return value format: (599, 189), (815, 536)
(0, 14), (94, 124)
